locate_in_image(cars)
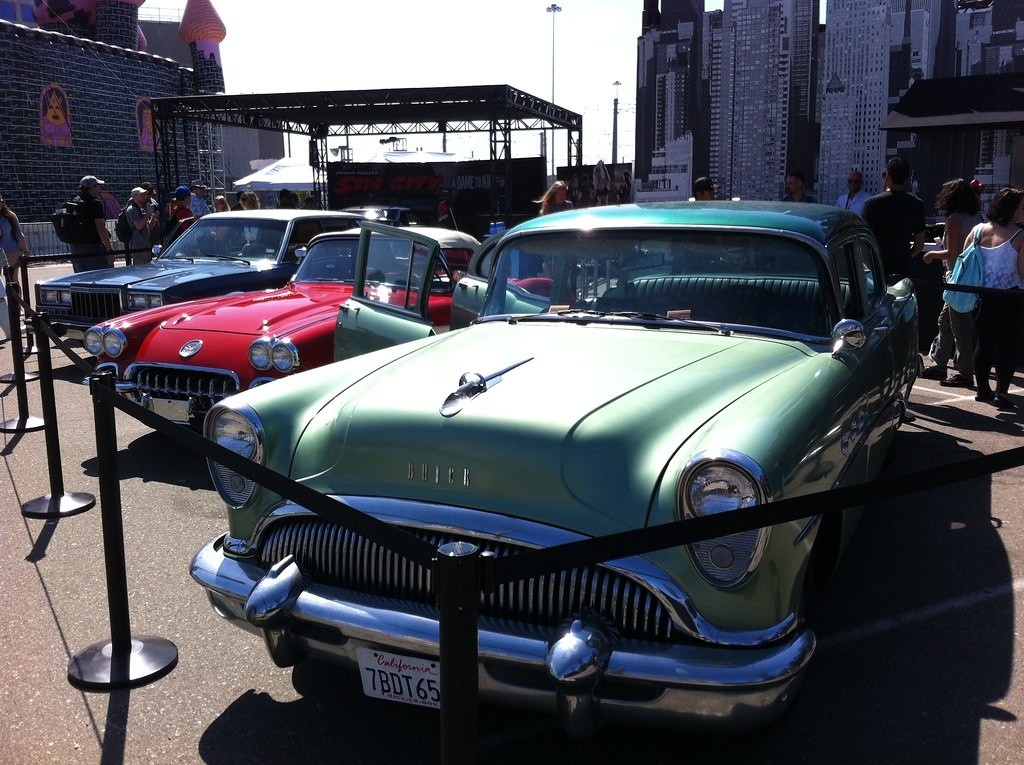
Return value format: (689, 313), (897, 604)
(338, 205), (431, 228)
(186, 193), (924, 741)
(83, 225), (575, 429)
(33, 207), (386, 341)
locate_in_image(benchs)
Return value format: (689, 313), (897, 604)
(624, 274), (856, 334)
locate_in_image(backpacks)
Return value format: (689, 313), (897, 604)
(50, 195), (97, 244)
(113, 201), (142, 244)
(942, 222), (986, 314)
(163, 208), (194, 247)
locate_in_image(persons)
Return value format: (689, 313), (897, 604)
(836, 157), (927, 281)
(70, 175), (115, 274)
(532, 181), (575, 300)
(962, 188), (1024, 408)
(0, 197), (30, 340)
(125, 180), (316, 266)
(918, 178), (985, 387)
(780, 171), (818, 203)
(573, 160), (632, 208)
(680, 177), (727, 260)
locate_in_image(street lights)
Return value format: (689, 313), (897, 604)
(546, 3), (562, 186)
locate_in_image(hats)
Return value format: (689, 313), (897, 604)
(279, 189), (292, 199)
(175, 186), (193, 201)
(691, 177), (722, 193)
(131, 187), (147, 197)
(191, 180), (207, 187)
(80, 176), (105, 188)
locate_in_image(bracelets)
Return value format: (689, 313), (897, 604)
(24, 251), (29, 253)
(108, 249), (113, 252)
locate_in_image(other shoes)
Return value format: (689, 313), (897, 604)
(974, 390), (993, 402)
(993, 392), (1015, 407)
(939, 374), (976, 389)
(919, 364), (948, 379)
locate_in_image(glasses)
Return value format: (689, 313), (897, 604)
(847, 178), (860, 184)
